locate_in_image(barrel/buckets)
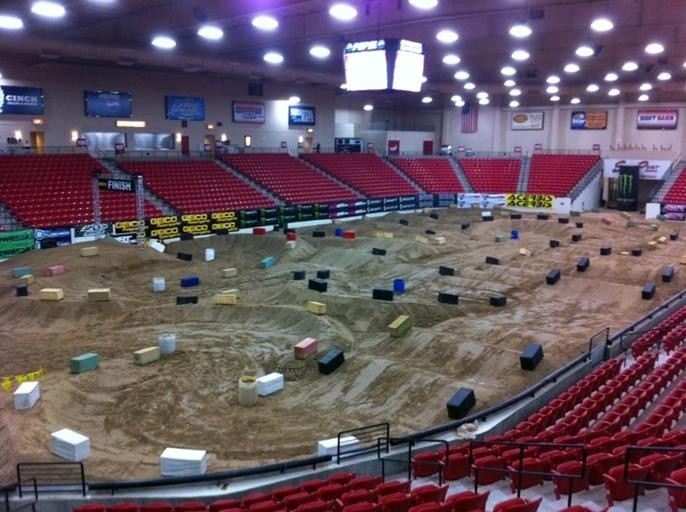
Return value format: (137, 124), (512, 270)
(617, 165), (639, 211)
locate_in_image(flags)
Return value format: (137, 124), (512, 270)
(459, 100), (480, 133)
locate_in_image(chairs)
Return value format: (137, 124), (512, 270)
(74, 467), (602, 511)
(409, 291), (685, 512)
(661, 162), (686, 206)
(0, 152), (602, 229)
